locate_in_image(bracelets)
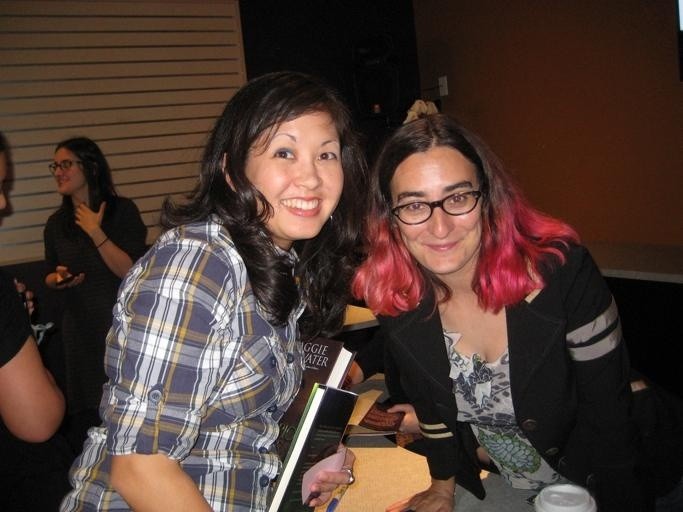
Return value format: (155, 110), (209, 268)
(95, 236), (109, 249)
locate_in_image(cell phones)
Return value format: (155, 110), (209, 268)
(56, 272), (76, 285)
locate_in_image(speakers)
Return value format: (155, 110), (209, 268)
(238, 0), (421, 134)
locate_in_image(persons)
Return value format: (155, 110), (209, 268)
(41, 136), (148, 402)
(342, 329), (421, 437)
(53, 67), (374, 512)
(0, 131), (69, 511)
(350, 113), (682, 512)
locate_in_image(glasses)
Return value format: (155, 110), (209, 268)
(49, 159), (82, 174)
(390, 188), (483, 226)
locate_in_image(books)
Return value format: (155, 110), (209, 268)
(348, 405), (405, 439)
(275, 335), (358, 461)
(268, 380), (358, 512)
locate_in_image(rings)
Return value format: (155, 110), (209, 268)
(404, 504), (416, 512)
(340, 468), (354, 485)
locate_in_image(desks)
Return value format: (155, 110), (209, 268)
(340, 304), (381, 334)
(314, 444), (490, 512)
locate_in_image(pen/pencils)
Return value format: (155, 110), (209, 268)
(326, 483), (348, 512)
